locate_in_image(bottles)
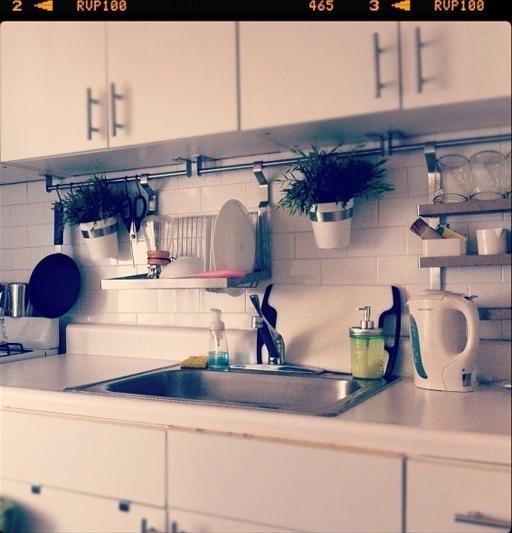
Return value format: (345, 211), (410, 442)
(6, 281), (30, 319)
(146, 250), (172, 278)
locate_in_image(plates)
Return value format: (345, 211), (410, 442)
(211, 198), (256, 277)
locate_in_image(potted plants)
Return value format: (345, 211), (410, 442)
(48, 172), (126, 264)
(270, 142), (395, 249)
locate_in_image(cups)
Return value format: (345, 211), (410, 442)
(432, 154), (470, 205)
(475, 227), (508, 256)
(468, 150), (509, 201)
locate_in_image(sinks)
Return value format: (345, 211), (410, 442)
(64, 361), (402, 417)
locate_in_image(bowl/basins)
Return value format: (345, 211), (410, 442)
(159, 254), (206, 278)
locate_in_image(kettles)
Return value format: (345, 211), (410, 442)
(406, 289), (480, 394)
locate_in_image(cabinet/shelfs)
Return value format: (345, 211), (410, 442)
(0, 21), (240, 179)
(1, 403), (512, 532)
(415, 198), (510, 274)
(235, 22), (512, 166)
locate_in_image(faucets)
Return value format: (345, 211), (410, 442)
(250, 294), (285, 365)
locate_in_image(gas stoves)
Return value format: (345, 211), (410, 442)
(0, 340), (34, 357)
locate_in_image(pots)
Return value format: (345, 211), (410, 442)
(26, 201), (82, 319)
